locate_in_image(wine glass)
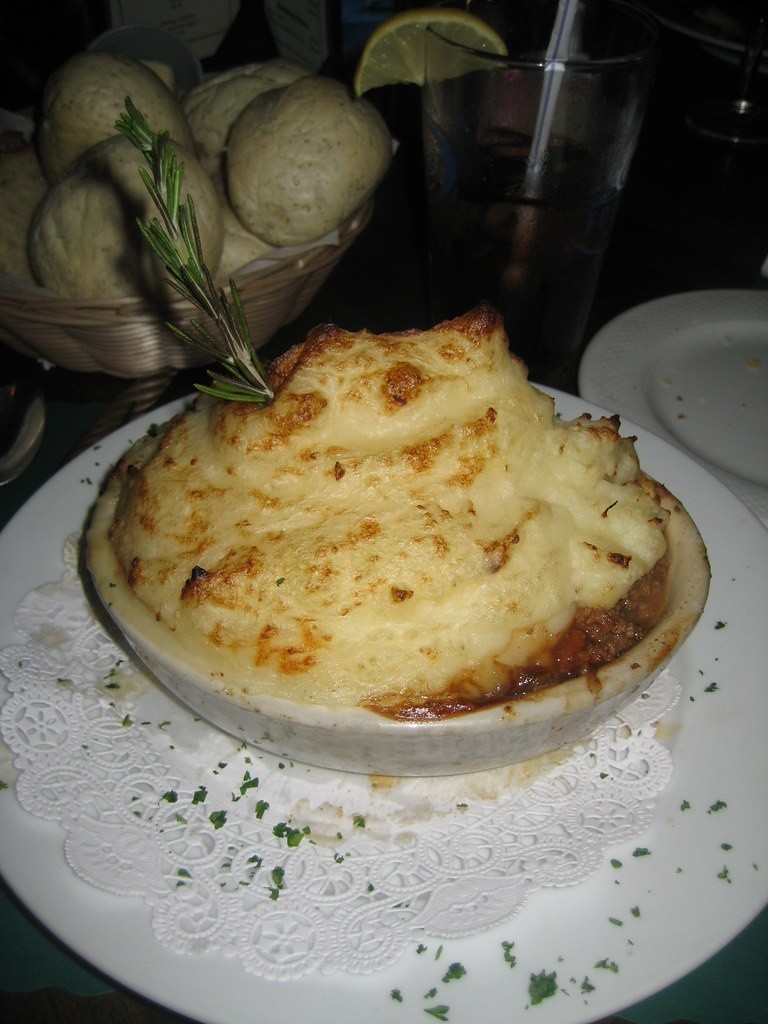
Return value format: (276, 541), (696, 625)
(683, 0), (768, 144)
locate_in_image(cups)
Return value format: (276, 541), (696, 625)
(419, 21), (652, 394)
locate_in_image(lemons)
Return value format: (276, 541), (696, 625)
(355, 14), (508, 94)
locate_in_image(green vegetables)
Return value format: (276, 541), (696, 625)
(113, 93), (277, 406)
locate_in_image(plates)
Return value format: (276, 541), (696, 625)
(634, 0), (768, 58)
(0, 381), (768, 1024)
(579, 289), (768, 525)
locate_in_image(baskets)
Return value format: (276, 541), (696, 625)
(0, 184), (375, 378)
